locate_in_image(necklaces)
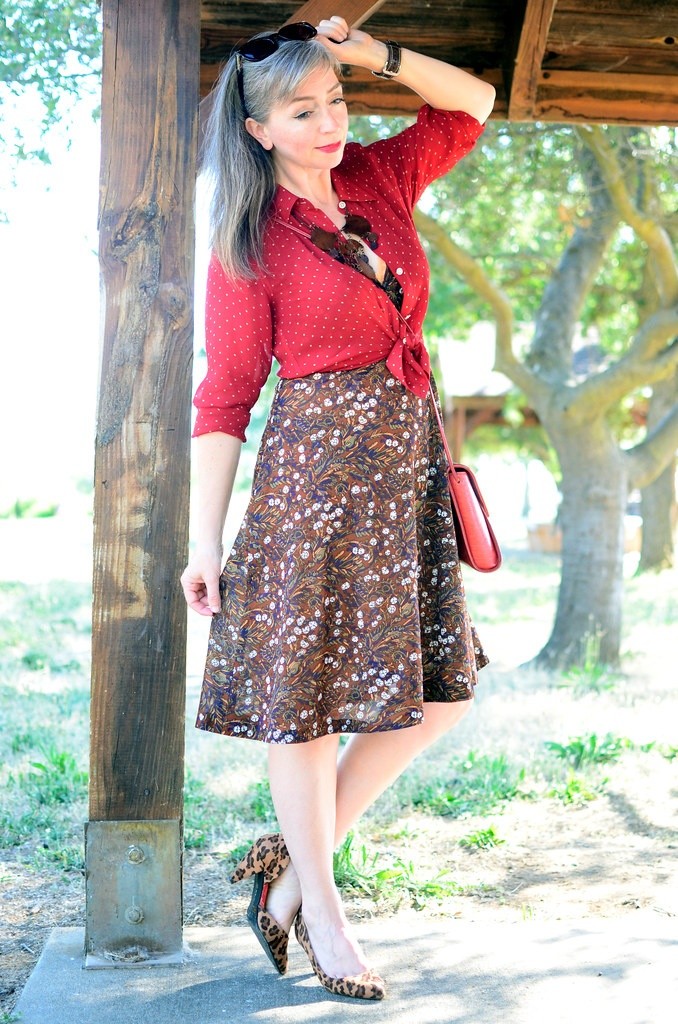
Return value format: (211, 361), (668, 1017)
(293, 202), (378, 278)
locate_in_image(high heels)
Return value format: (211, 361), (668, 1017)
(294, 903), (385, 1001)
(230, 832), (290, 975)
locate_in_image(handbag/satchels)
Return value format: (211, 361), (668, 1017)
(446, 458), (502, 573)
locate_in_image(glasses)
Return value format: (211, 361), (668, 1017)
(234, 21), (319, 124)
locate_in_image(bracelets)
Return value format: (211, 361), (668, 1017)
(371, 40), (402, 79)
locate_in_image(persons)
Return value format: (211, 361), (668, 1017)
(180, 16), (495, 1001)
